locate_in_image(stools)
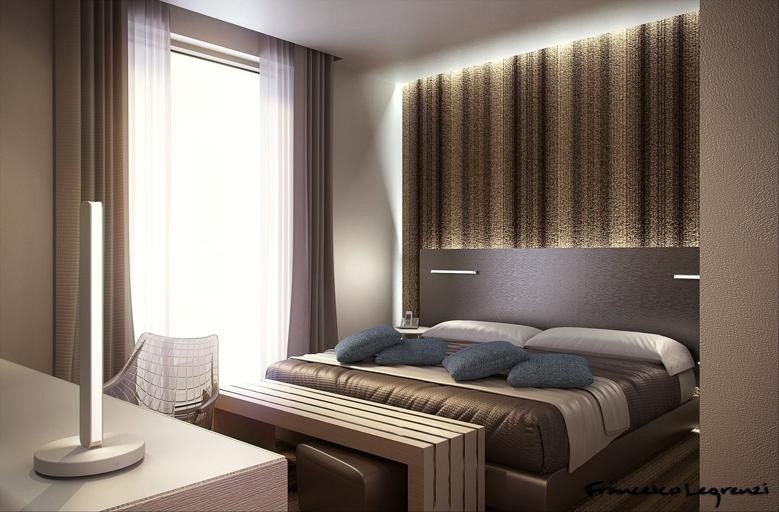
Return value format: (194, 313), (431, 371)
(295, 437), (408, 512)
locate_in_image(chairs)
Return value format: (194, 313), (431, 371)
(102, 331), (220, 430)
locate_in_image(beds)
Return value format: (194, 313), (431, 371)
(265, 247), (700, 512)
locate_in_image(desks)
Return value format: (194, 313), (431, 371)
(0, 359), (288, 512)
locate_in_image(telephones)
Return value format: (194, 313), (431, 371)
(400, 311), (419, 329)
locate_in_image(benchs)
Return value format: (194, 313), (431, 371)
(213, 378), (486, 512)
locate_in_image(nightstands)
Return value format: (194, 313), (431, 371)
(395, 326), (430, 339)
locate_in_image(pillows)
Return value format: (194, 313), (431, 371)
(374, 338), (448, 366)
(420, 319), (544, 349)
(334, 324), (403, 364)
(523, 326), (696, 377)
(506, 351), (595, 388)
(441, 341), (531, 382)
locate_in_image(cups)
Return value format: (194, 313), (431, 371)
(400, 311), (419, 328)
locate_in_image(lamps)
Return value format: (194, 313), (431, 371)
(34, 200), (145, 477)
